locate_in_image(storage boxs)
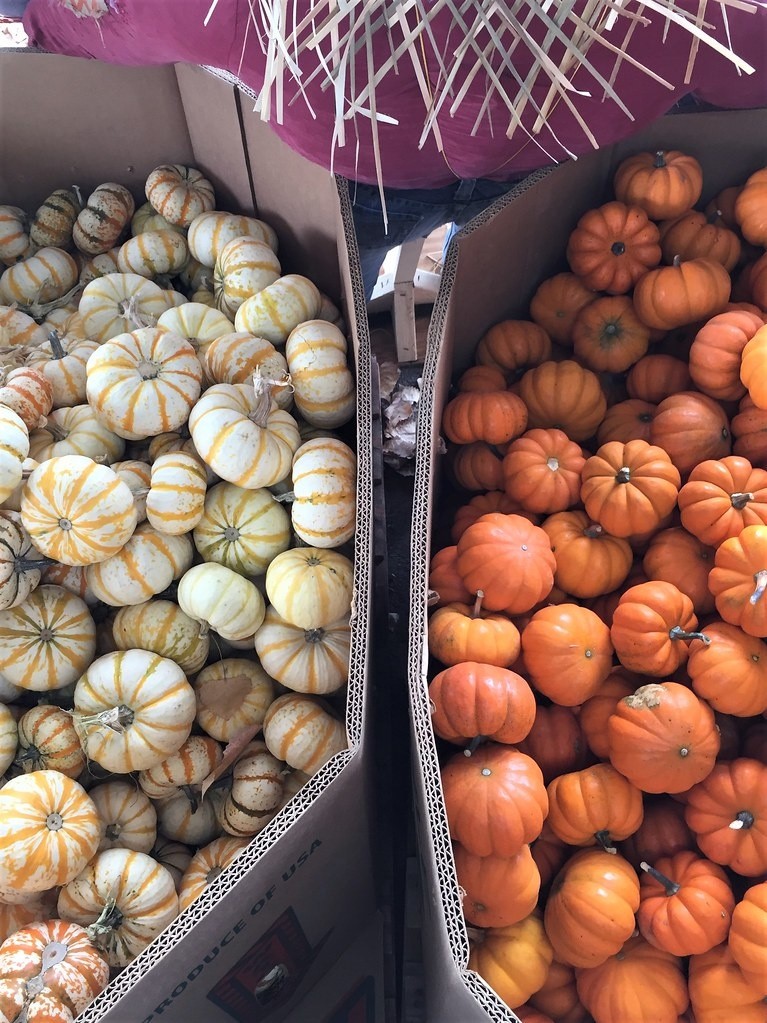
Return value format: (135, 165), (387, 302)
(367, 221), (458, 367)
(0, 46), (385, 1023)
(407, 106), (767, 1023)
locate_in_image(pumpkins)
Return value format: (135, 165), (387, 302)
(0, 166), (358, 1023)
(427, 146), (767, 1023)
